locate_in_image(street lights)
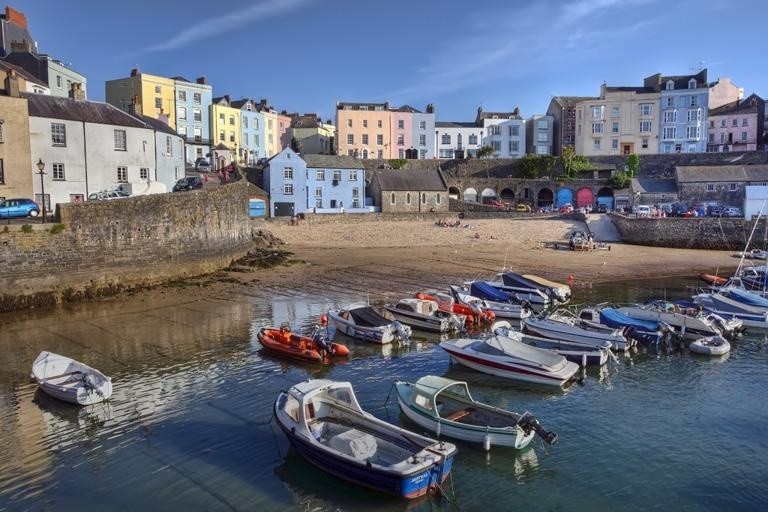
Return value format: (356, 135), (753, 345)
(231, 141), (237, 177)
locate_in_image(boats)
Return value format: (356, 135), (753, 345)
(271, 377), (458, 500)
(28, 349), (115, 406)
(322, 270), (571, 344)
(393, 373), (558, 450)
(257, 328), (350, 366)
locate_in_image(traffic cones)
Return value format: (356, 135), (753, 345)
(204, 174), (208, 181)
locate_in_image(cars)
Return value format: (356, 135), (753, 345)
(88, 191), (130, 201)
(0, 197), (39, 219)
(35, 157), (48, 222)
(495, 201), (612, 215)
(633, 200), (742, 218)
(171, 175), (202, 192)
(193, 157), (211, 172)
